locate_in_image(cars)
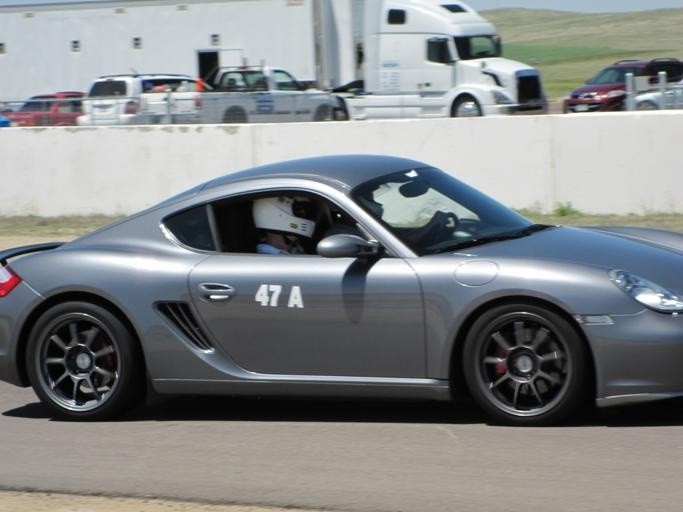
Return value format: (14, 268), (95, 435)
(621, 79), (683, 110)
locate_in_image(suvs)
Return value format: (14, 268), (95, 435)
(562, 53), (681, 114)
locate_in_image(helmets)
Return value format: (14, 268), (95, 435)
(252, 196), (316, 238)
(329, 177), (383, 216)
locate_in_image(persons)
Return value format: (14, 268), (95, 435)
(323, 183), (451, 249)
(0, 108), (13, 127)
(252, 191), (316, 254)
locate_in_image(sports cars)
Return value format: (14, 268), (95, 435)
(0, 153), (683, 430)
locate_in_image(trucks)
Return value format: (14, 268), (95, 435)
(0, 0), (550, 124)
